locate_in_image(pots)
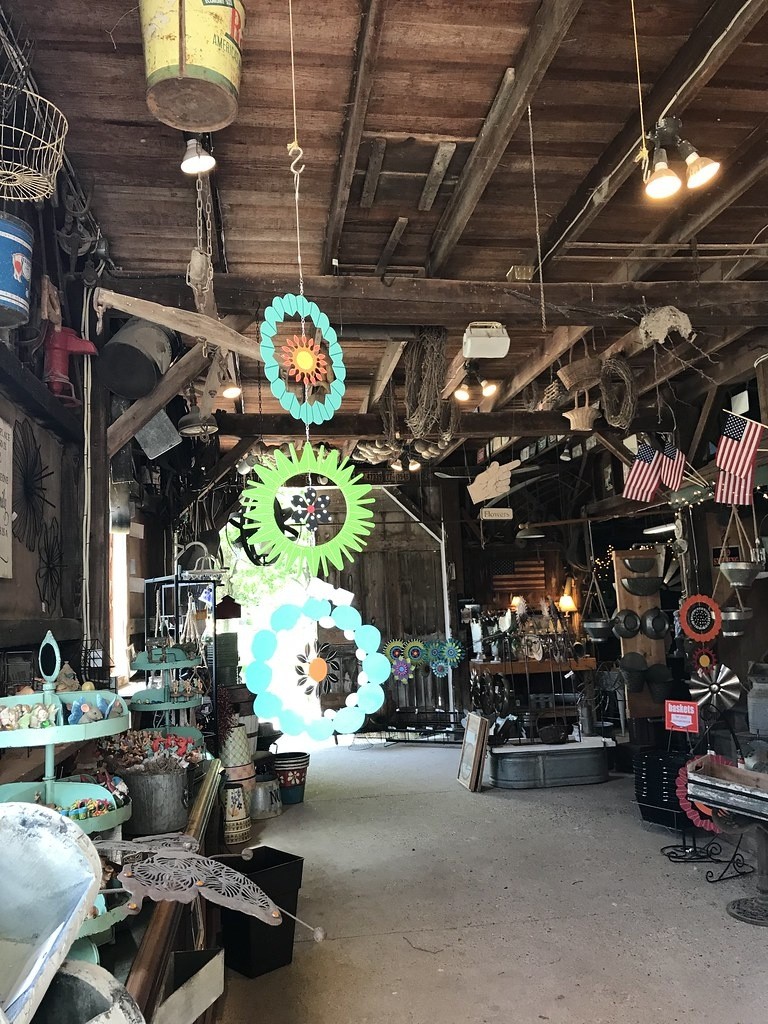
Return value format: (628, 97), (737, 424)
(587, 635), (617, 662)
(594, 722), (613, 737)
(641, 606), (670, 639)
(615, 609), (640, 638)
(581, 617), (614, 638)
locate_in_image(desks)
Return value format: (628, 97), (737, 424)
(65, 759), (223, 1024)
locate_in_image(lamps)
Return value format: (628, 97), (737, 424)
(644, 116), (721, 201)
(454, 359), (497, 401)
(511, 596), (521, 617)
(176, 379), (219, 437)
(222, 379), (240, 399)
(514, 463), (546, 538)
(558, 594), (578, 627)
(180, 130), (217, 176)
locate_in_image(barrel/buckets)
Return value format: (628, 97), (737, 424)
(96, 319), (180, 399)
(138, 0), (246, 134)
(122, 767), (189, 835)
(0, 210), (35, 330)
(627, 717), (670, 747)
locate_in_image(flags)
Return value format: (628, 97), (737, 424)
(660, 441), (686, 493)
(716, 414), (763, 480)
(622, 443), (663, 502)
(714, 463), (754, 505)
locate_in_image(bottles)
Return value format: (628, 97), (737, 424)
(747, 684), (768, 735)
